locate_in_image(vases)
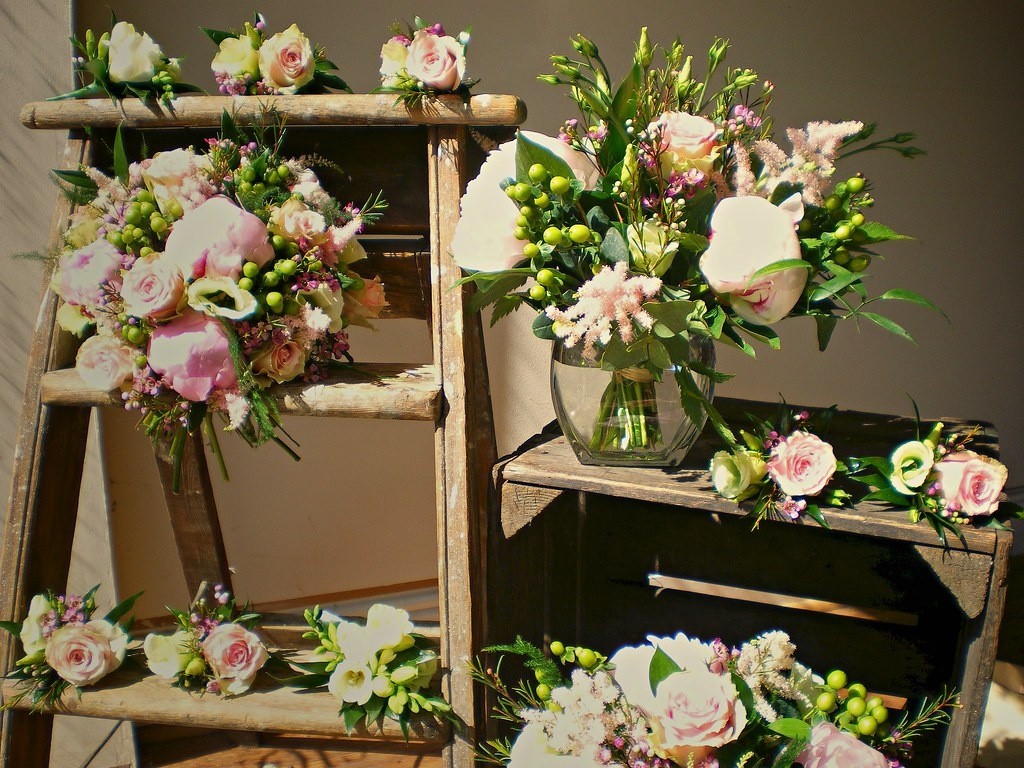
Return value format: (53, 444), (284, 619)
(549, 332), (716, 471)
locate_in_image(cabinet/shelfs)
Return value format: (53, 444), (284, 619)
(3, 89), (532, 766)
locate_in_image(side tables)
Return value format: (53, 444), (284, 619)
(487, 394), (1019, 768)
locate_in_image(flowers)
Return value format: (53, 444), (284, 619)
(38, 16), (203, 113)
(476, 628), (962, 768)
(44, 104), (388, 495)
(0, 585), (141, 714)
(207, 15), (361, 99)
(450, 27), (956, 458)
(297, 600), (449, 738)
(145, 581), (302, 699)
(697, 398), (858, 543)
(855, 393), (1008, 548)
(373, 15), (483, 113)
